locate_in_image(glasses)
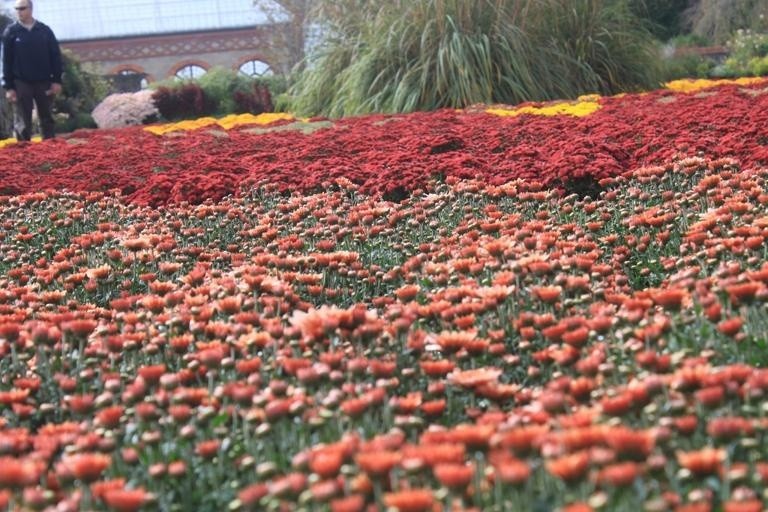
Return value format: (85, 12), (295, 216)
(16, 7), (27, 10)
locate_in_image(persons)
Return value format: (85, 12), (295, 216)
(0, 0), (64, 143)
